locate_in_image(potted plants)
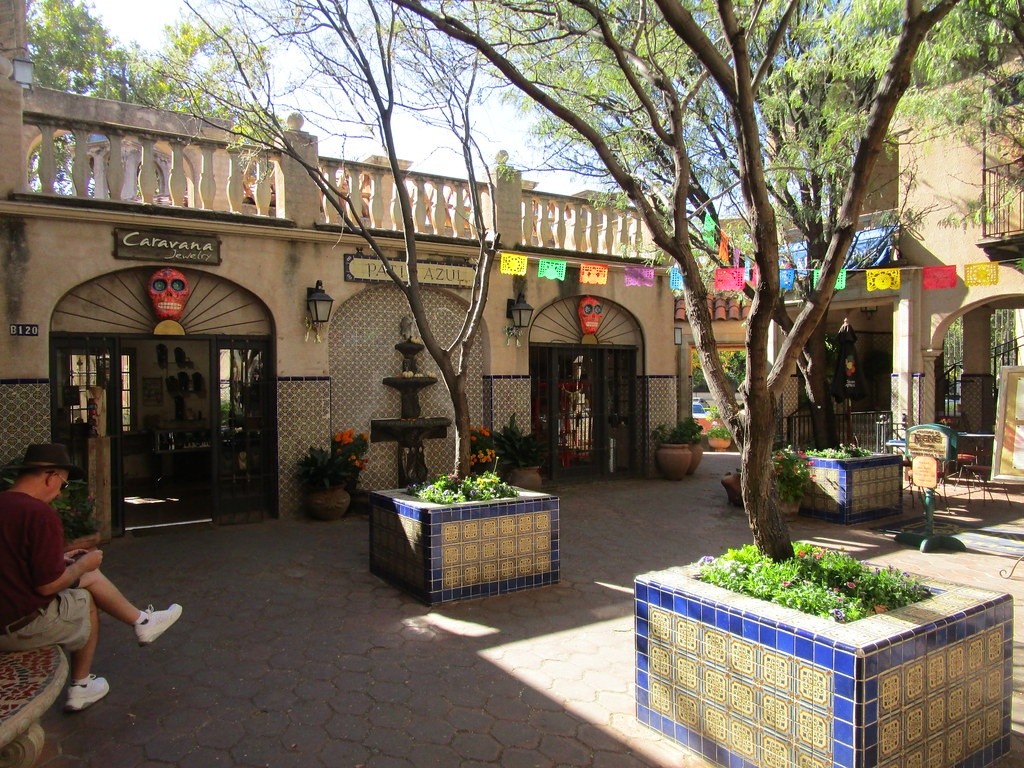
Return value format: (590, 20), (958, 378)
(656, 418), (704, 482)
(298, 449), (351, 520)
(707, 426), (732, 452)
(494, 413), (551, 491)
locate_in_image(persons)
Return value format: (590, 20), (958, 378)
(0, 442), (183, 713)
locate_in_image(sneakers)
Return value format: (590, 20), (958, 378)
(134, 603), (183, 647)
(65, 673), (109, 710)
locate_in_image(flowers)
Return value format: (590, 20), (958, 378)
(0, 458), (98, 540)
(469, 425), (495, 463)
(332, 431), (370, 483)
(771, 444), (815, 500)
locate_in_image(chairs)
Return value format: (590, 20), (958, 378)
(901, 442), (1013, 515)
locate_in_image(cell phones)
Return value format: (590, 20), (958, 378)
(70, 552), (87, 565)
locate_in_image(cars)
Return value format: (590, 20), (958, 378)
(692, 401), (709, 419)
(693, 397), (710, 408)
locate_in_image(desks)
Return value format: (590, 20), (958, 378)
(958, 433), (995, 480)
(153, 426), (211, 483)
(885, 440), (906, 448)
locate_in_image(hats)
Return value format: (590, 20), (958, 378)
(0, 444), (84, 480)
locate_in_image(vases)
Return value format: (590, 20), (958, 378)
(333, 468), (360, 513)
(785, 500), (799, 521)
(471, 463), (493, 475)
(63, 532), (101, 556)
(721, 472), (743, 508)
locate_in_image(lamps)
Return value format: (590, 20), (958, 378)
(306, 279), (334, 323)
(505, 290), (534, 327)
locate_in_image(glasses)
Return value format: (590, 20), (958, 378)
(46, 470), (70, 490)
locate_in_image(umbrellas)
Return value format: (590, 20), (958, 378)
(829, 318), (871, 444)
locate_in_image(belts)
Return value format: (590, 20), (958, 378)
(0, 603), (49, 635)
